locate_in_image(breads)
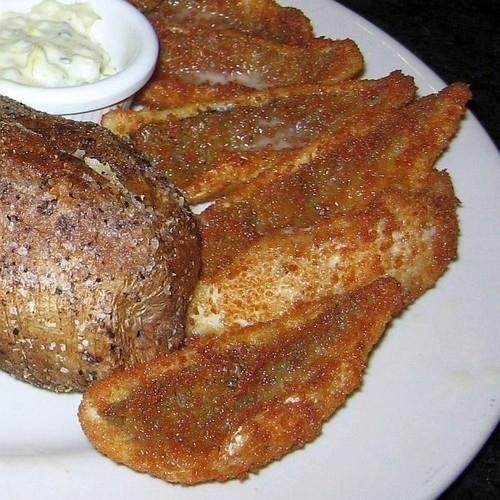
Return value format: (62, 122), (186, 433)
(0, 94), (201, 394)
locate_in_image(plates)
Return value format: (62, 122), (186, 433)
(0, 1), (500, 496)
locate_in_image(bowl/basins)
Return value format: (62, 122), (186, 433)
(0, 1), (159, 126)
